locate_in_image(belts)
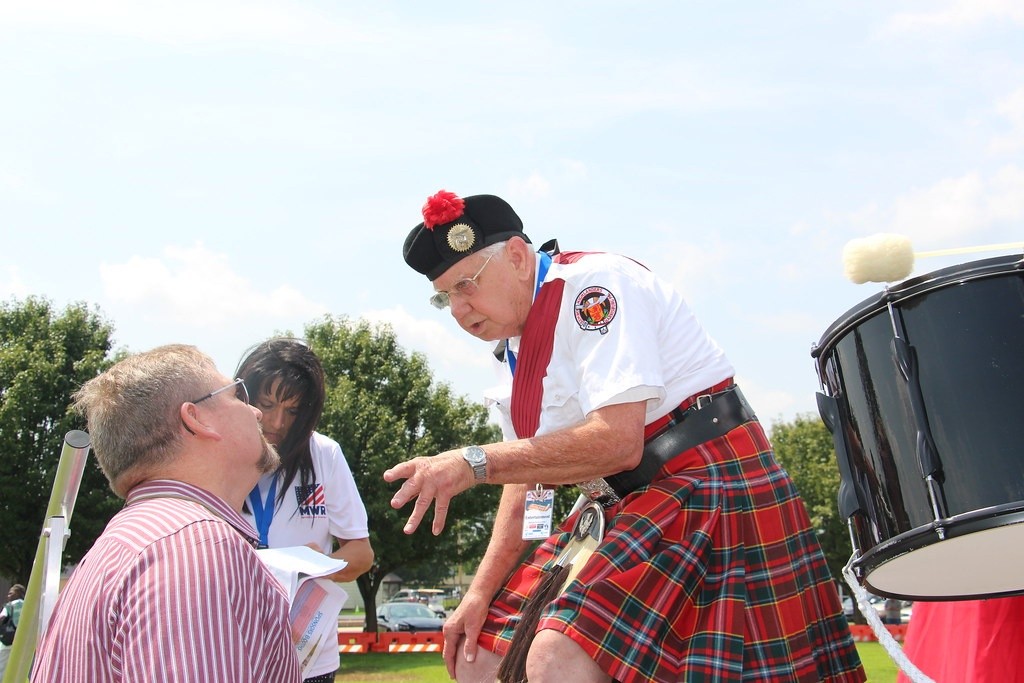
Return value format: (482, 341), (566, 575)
(577, 382), (758, 510)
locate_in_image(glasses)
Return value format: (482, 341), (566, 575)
(181, 377), (251, 437)
(429, 249), (500, 310)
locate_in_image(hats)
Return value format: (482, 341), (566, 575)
(13, 584), (25, 598)
(404, 189), (525, 282)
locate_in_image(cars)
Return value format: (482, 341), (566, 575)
(840, 594), (912, 623)
(386, 589), (428, 606)
(362, 602), (443, 631)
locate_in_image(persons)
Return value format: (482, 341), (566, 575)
(0, 585), (26, 646)
(233, 337), (374, 683)
(31, 344), (302, 683)
(383, 189), (867, 683)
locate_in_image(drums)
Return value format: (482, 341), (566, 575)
(810, 254), (1024, 603)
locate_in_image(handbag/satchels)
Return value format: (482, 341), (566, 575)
(0, 616), (17, 646)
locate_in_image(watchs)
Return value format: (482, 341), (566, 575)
(462, 446), (488, 484)
